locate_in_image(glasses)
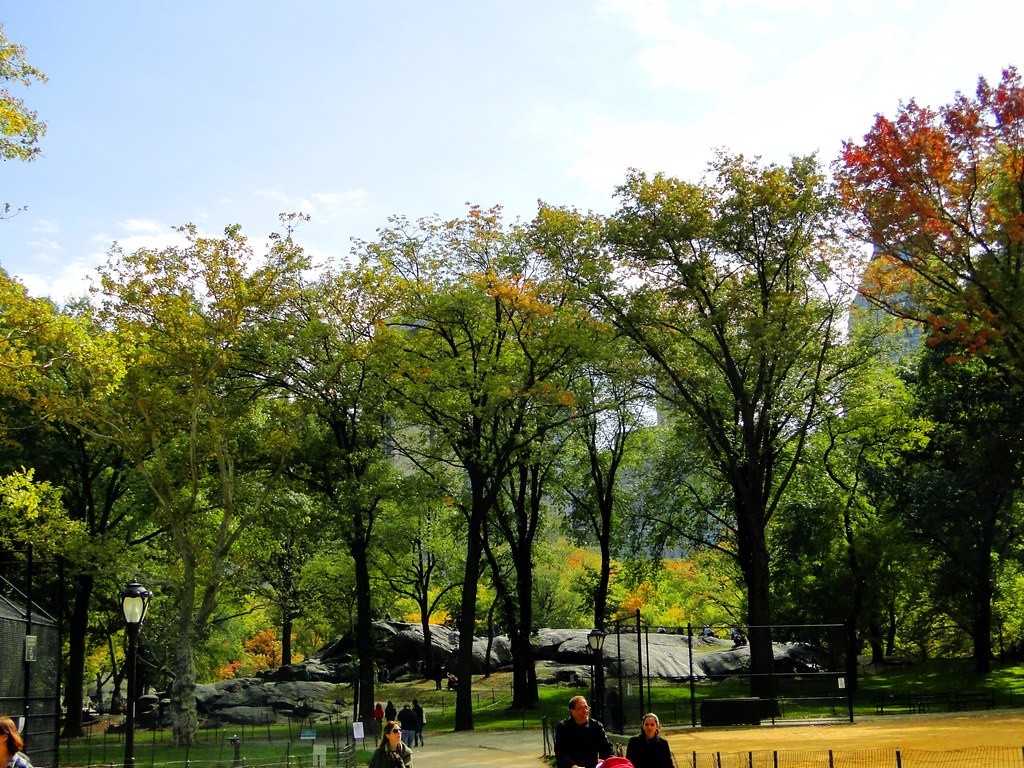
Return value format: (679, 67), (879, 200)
(574, 706), (591, 710)
(390, 729), (403, 733)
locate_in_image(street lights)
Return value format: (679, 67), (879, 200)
(587, 628), (604, 725)
(118, 577), (153, 768)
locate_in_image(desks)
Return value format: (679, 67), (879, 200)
(699, 696), (782, 726)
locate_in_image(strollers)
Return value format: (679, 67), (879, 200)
(595, 755), (634, 768)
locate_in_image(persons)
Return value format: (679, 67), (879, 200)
(609, 622), (747, 646)
(625, 713), (674, 768)
(374, 699), (424, 748)
(373, 661), (458, 691)
(369, 721), (415, 768)
(554, 696), (616, 768)
(0, 716), (33, 768)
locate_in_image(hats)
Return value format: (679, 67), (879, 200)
(411, 699), (419, 704)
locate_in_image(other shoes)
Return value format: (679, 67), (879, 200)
(414, 742), (418, 748)
(421, 740), (424, 747)
